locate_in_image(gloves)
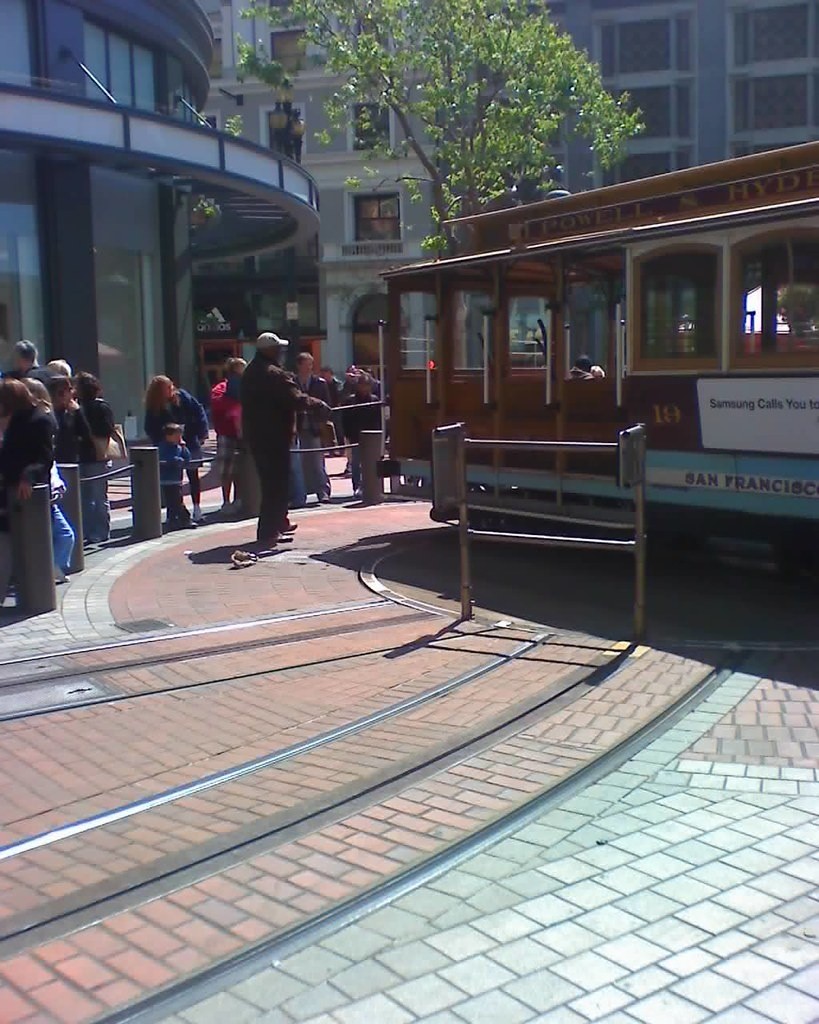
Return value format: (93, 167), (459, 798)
(318, 401), (332, 415)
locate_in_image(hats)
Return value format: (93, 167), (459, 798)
(256, 332), (289, 355)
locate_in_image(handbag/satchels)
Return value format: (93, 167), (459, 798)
(80, 406), (128, 462)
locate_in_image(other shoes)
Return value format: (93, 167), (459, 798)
(193, 510), (201, 522)
(178, 520), (198, 528)
(89, 535), (109, 543)
(54, 564), (70, 583)
(280, 522), (297, 533)
(344, 469), (353, 477)
(277, 535), (292, 543)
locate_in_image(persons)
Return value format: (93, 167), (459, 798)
(240, 332), (337, 543)
(0, 339), (113, 607)
(211, 358), (253, 513)
(567, 355), (606, 380)
(280, 352), (391, 510)
(144, 374), (210, 529)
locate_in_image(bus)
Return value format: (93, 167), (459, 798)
(377, 140), (819, 551)
